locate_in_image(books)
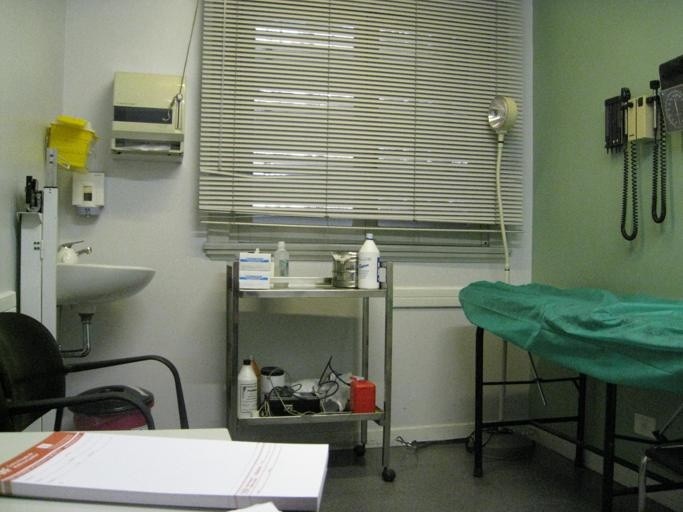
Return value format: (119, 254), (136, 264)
(0, 427), (331, 512)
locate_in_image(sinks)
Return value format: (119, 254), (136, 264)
(56, 262), (157, 305)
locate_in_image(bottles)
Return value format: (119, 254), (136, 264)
(238, 355), (286, 421)
(274, 241), (288, 290)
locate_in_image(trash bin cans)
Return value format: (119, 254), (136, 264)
(67, 385), (155, 430)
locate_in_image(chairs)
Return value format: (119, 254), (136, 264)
(0, 313), (189, 432)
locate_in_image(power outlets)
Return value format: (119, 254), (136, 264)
(632, 413), (655, 439)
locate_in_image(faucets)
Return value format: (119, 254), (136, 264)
(61, 239), (92, 261)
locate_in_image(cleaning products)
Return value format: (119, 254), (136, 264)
(237, 354), (260, 420)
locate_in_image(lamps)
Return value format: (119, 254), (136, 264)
(466, 95), (536, 462)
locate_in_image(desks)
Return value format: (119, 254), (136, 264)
(0, 428), (232, 512)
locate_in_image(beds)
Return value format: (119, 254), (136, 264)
(458, 281), (683, 512)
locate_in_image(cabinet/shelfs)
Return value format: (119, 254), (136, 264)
(224, 257), (393, 484)
